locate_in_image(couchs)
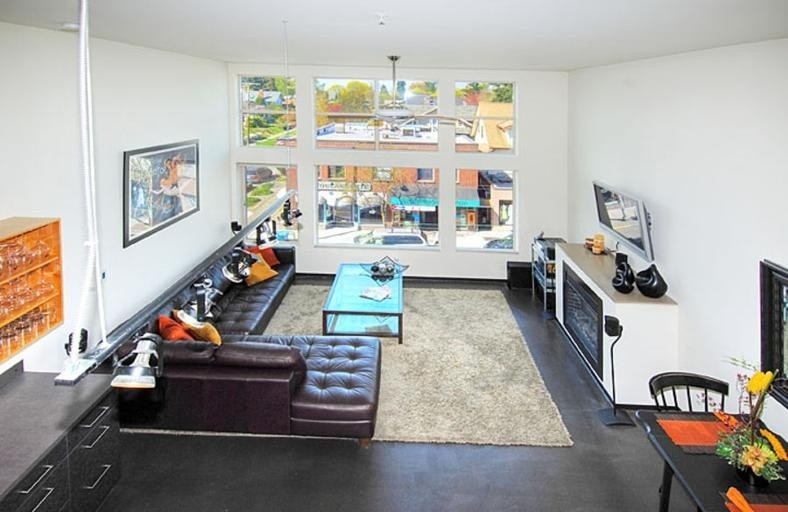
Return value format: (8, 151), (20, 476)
(123, 332), (382, 435)
(134, 240), (296, 343)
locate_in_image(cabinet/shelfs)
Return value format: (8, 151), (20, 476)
(71, 391), (134, 512)
(0, 219), (64, 365)
(530, 238), (567, 315)
(2, 431), (68, 511)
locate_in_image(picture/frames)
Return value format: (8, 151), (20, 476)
(759, 259), (788, 403)
(122, 138), (202, 249)
(716, 368), (788, 483)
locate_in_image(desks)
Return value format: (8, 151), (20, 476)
(636, 410), (788, 511)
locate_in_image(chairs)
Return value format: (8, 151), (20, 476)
(650, 366), (730, 415)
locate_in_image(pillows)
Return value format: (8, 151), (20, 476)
(241, 246), (278, 286)
(159, 303), (226, 345)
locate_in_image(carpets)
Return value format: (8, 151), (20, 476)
(260, 286), (576, 447)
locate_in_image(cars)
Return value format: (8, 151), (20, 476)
(353, 229), (427, 244)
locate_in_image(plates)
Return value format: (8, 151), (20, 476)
(360, 256), (410, 276)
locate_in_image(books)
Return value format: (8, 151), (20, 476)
(359, 286), (391, 302)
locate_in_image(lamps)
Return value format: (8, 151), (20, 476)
(190, 273), (224, 322)
(375, 53), (415, 131)
(281, 199), (302, 227)
(256, 216), (278, 248)
(223, 247), (259, 286)
(597, 315), (637, 426)
(111, 333), (161, 392)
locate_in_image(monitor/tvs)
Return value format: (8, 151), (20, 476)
(592, 179), (654, 263)
